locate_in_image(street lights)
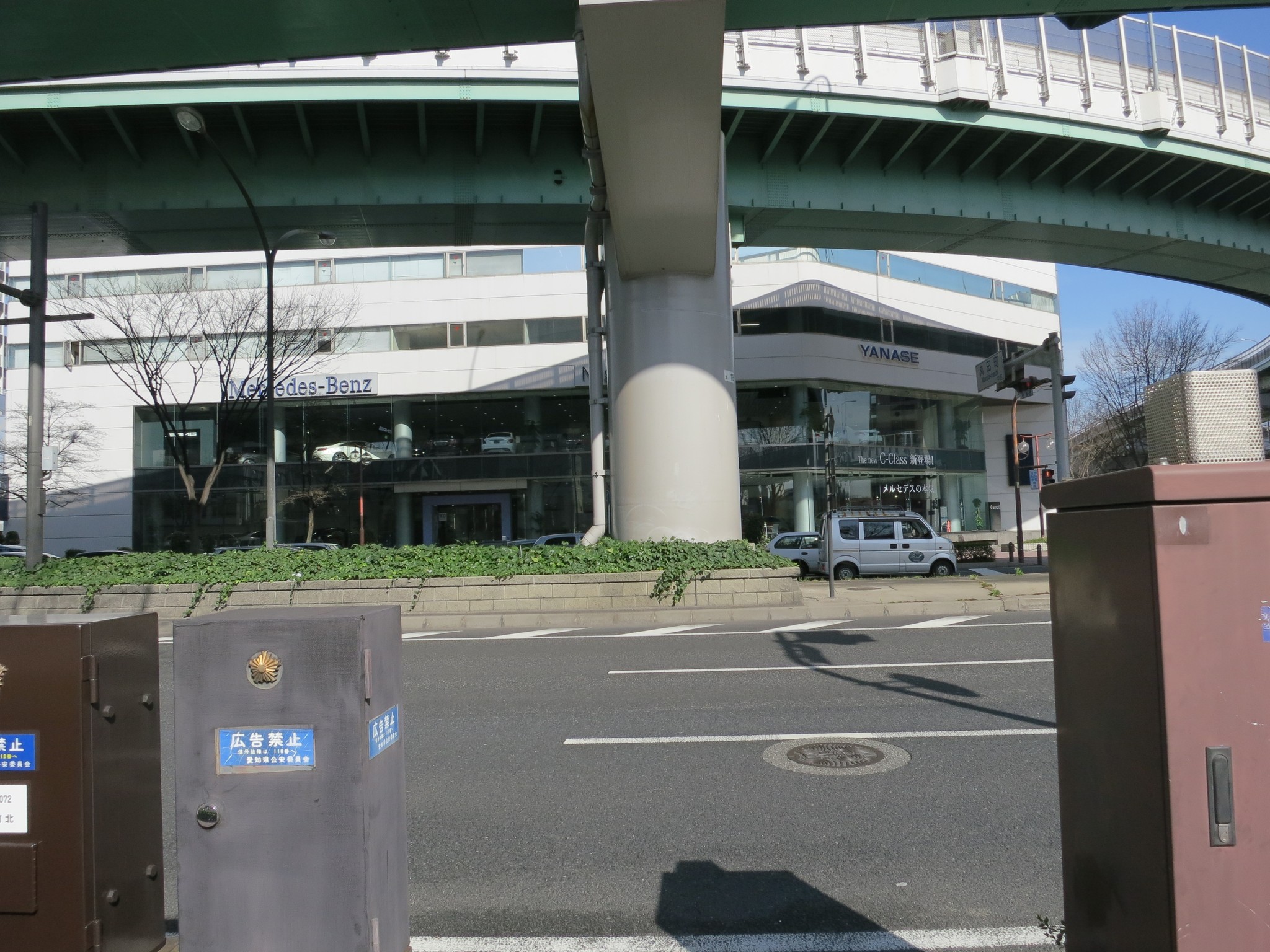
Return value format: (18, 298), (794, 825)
(175, 106), (336, 549)
(1018, 432), (1056, 537)
(348, 441), (374, 545)
(841, 399), (856, 438)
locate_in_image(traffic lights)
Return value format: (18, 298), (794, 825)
(1008, 376), (1034, 392)
(1060, 374), (1077, 403)
(1042, 468), (1055, 485)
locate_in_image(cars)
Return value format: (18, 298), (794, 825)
(480, 431), (517, 454)
(311, 440), (395, 459)
(766, 531), (823, 579)
(815, 429), (841, 443)
(845, 426), (885, 445)
(426, 430), (463, 455)
(74, 549), (140, 559)
(476, 533), (587, 552)
(0, 544), (57, 560)
(235, 447), (304, 463)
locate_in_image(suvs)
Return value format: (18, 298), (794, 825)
(211, 542), (342, 555)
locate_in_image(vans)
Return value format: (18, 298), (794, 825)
(817, 505), (957, 581)
